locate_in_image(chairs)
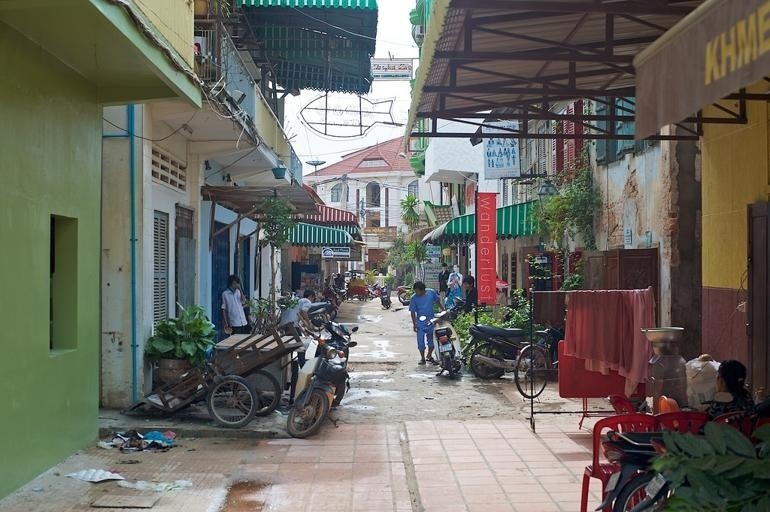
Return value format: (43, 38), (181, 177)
(580, 393), (769, 512)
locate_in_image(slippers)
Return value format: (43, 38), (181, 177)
(418, 359), (426, 364)
(425, 355), (434, 362)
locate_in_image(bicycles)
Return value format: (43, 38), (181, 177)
(511, 327), (566, 403)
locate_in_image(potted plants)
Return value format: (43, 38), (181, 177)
(144, 302), (216, 383)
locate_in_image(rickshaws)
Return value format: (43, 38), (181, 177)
(116, 321), (303, 430)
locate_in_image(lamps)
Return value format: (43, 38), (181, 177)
(228, 87), (248, 108)
(503, 171), (561, 214)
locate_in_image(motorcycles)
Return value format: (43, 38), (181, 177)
(364, 283), (412, 309)
(287, 288), (359, 438)
(595, 427), (702, 512)
(461, 321), (565, 380)
(418, 297), (467, 377)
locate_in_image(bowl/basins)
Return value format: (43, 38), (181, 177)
(641, 327), (686, 343)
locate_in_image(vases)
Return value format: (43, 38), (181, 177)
(270, 168), (287, 180)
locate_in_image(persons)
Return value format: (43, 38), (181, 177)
(407, 281), (446, 365)
(698, 356), (758, 424)
(437, 262), (452, 311)
(333, 273), (341, 287)
(339, 275), (349, 290)
(462, 274), (478, 315)
(279, 288), (316, 344)
(220, 275), (250, 335)
(442, 264), (463, 312)
